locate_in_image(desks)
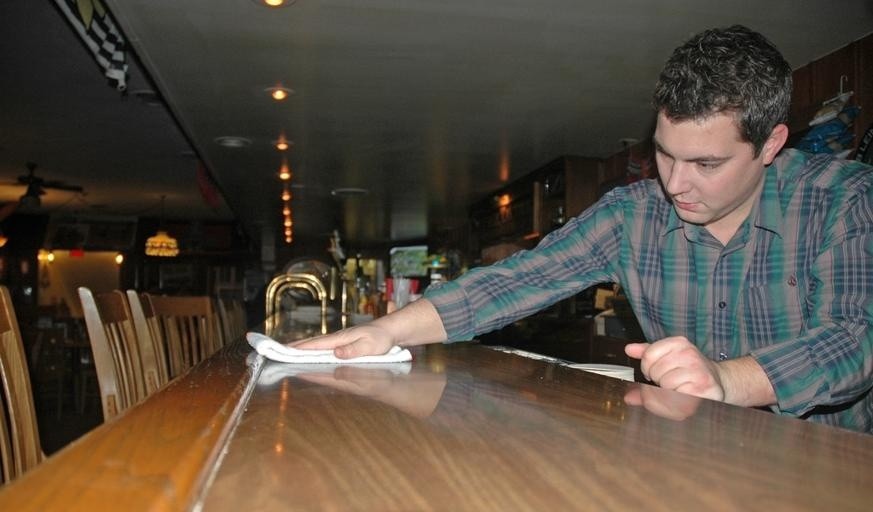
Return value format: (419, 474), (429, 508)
(1, 307), (873, 512)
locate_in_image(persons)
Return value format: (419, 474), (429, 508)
(282, 24), (872, 437)
(294, 367), (702, 512)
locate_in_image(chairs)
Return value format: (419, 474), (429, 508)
(0, 286), (49, 486)
(61, 281), (248, 427)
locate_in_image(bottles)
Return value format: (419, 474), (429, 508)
(347, 273), (442, 319)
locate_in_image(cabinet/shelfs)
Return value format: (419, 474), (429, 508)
(790, 33), (873, 159)
(468, 134), (655, 267)
